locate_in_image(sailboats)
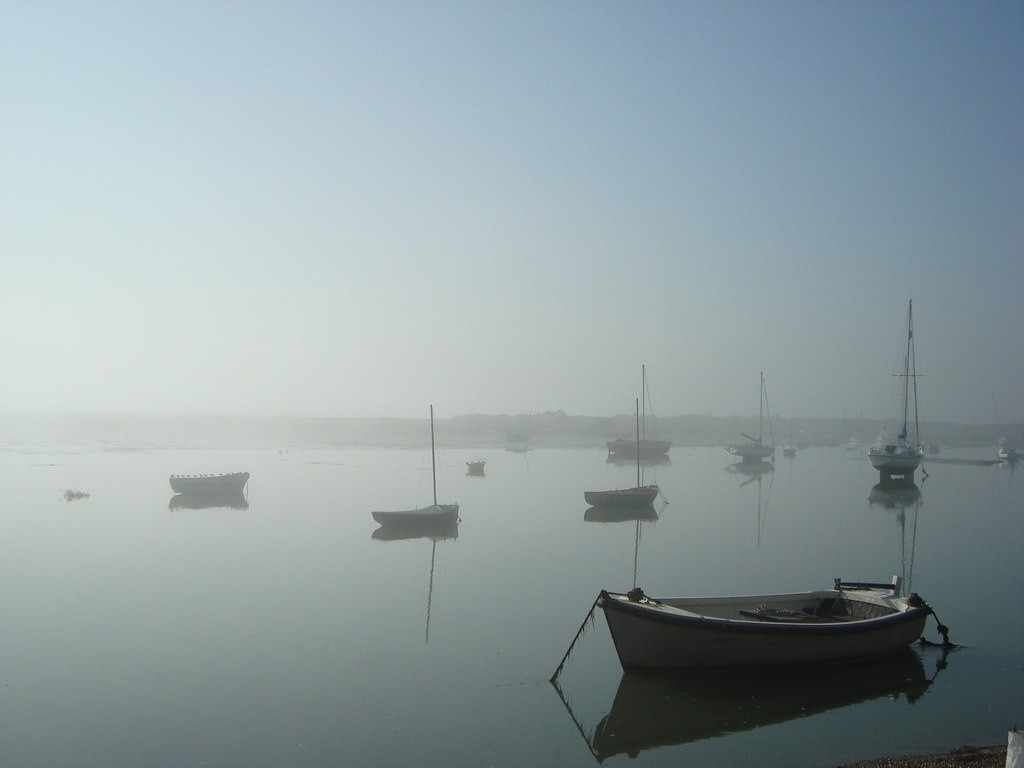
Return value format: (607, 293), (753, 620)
(369, 524), (460, 642)
(583, 506), (660, 593)
(368, 405), (463, 528)
(584, 399), (663, 506)
(784, 453), (797, 482)
(604, 362), (673, 456)
(867, 476), (923, 582)
(719, 367), (780, 461)
(606, 454), (672, 484)
(865, 297), (928, 475)
(726, 458), (777, 549)
(782, 421), (799, 453)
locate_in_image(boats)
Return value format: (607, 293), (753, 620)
(600, 572), (939, 670)
(167, 492), (251, 514)
(169, 471), (251, 494)
(999, 448), (1023, 459)
(589, 645), (929, 760)
(464, 459), (488, 479)
